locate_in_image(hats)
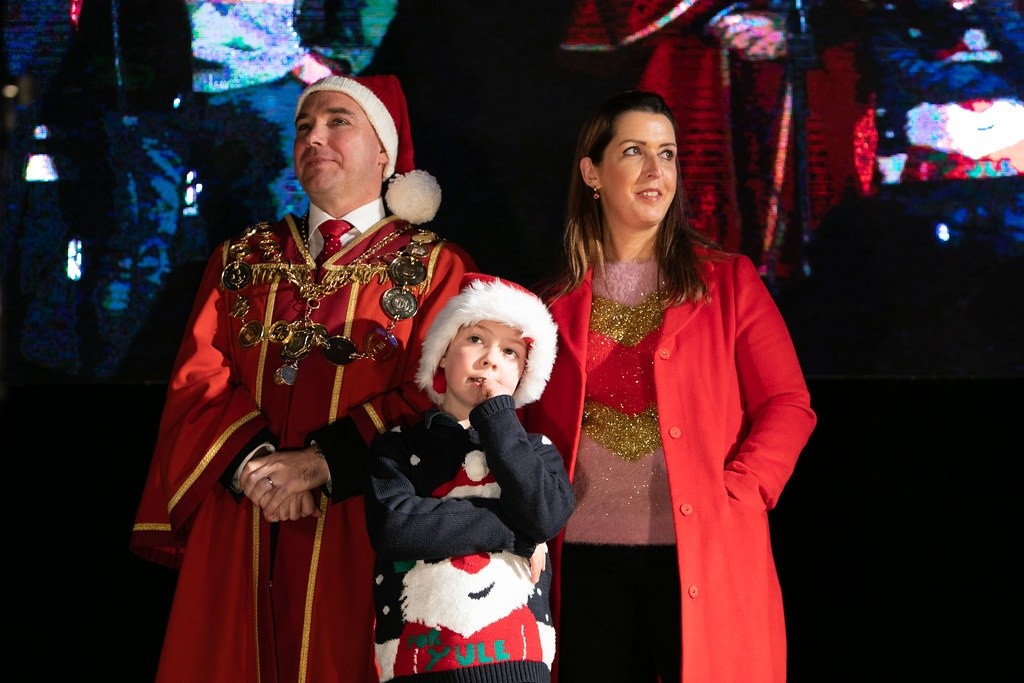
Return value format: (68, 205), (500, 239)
(294, 73), (442, 224)
(416, 272), (561, 411)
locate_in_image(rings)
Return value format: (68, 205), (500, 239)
(265, 476), (276, 488)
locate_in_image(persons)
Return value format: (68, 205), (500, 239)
(362, 273), (571, 683)
(161, 76), (475, 683)
(0, 0), (1024, 373)
(501, 91), (816, 683)
